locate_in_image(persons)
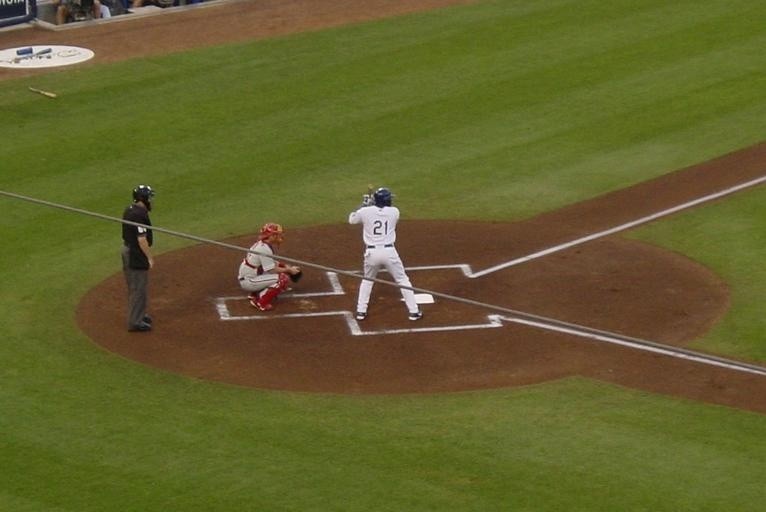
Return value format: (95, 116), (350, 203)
(52, 0), (101, 25)
(348, 183), (424, 322)
(237, 222), (302, 313)
(125, 0), (160, 8)
(119, 184), (152, 332)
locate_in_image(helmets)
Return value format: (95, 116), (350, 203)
(133, 185), (157, 197)
(260, 223), (285, 240)
(375, 187), (392, 201)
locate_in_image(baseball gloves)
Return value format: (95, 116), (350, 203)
(288, 271), (302, 282)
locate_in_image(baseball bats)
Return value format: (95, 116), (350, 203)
(16, 48), (52, 59)
(367, 184), (373, 198)
(28, 87), (57, 98)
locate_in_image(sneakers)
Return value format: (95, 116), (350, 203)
(408, 312), (423, 320)
(247, 294), (274, 311)
(127, 315), (152, 332)
(355, 312), (367, 321)
